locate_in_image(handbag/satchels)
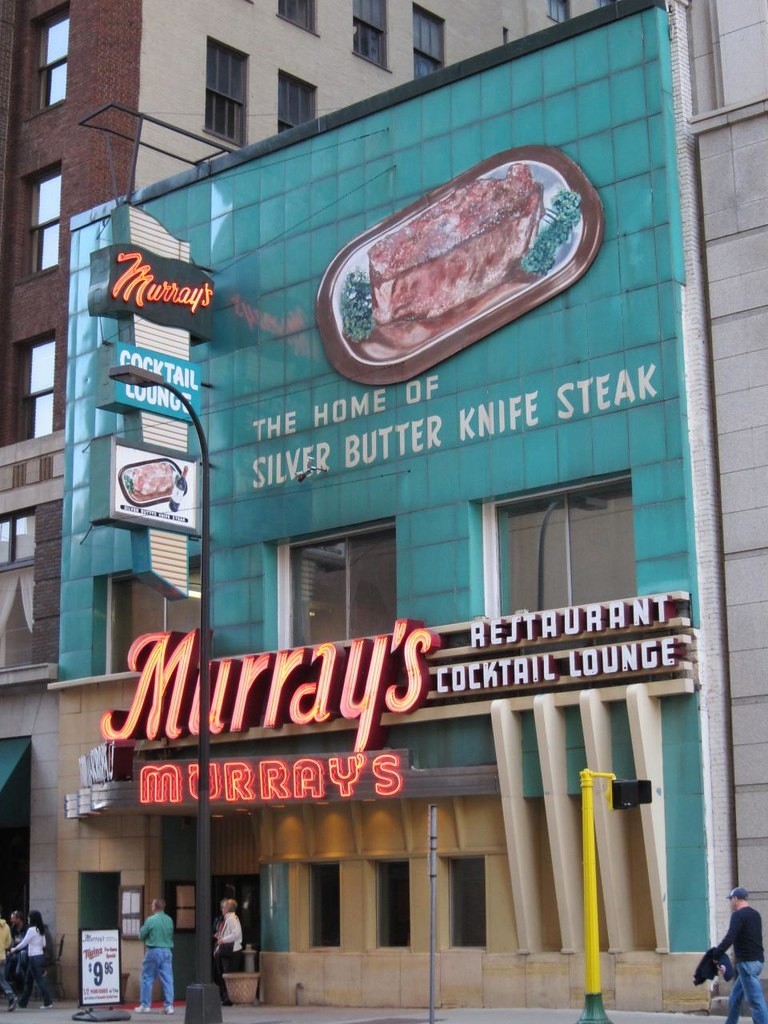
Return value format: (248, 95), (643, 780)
(213, 939), (234, 959)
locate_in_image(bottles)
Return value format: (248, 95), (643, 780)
(169, 466), (189, 512)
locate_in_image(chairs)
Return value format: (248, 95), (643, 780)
(33, 935), (65, 1002)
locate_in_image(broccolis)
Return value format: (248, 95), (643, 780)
(522, 190), (584, 277)
(340, 270), (375, 341)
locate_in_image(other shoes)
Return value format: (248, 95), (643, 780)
(5, 996), (19, 1011)
(134, 1005), (150, 1013)
(160, 1005), (174, 1015)
(39, 1004), (53, 1009)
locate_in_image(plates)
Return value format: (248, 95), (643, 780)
(118, 459), (187, 507)
(314, 145), (605, 386)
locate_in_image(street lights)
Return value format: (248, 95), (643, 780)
(534, 493), (609, 614)
(106, 365), (223, 1024)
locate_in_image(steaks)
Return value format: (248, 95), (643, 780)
(368, 164), (544, 324)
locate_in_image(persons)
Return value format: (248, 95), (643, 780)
(134, 898), (174, 1014)
(212, 898), (243, 1006)
(0, 905), (18, 1012)
(713, 887), (768, 1024)
(10, 910), (53, 1009)
(5, 910), (29, 994)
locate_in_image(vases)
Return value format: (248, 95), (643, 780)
(223, 972), (262, 1007)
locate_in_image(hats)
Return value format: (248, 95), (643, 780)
(725, 887), (748, 900)
